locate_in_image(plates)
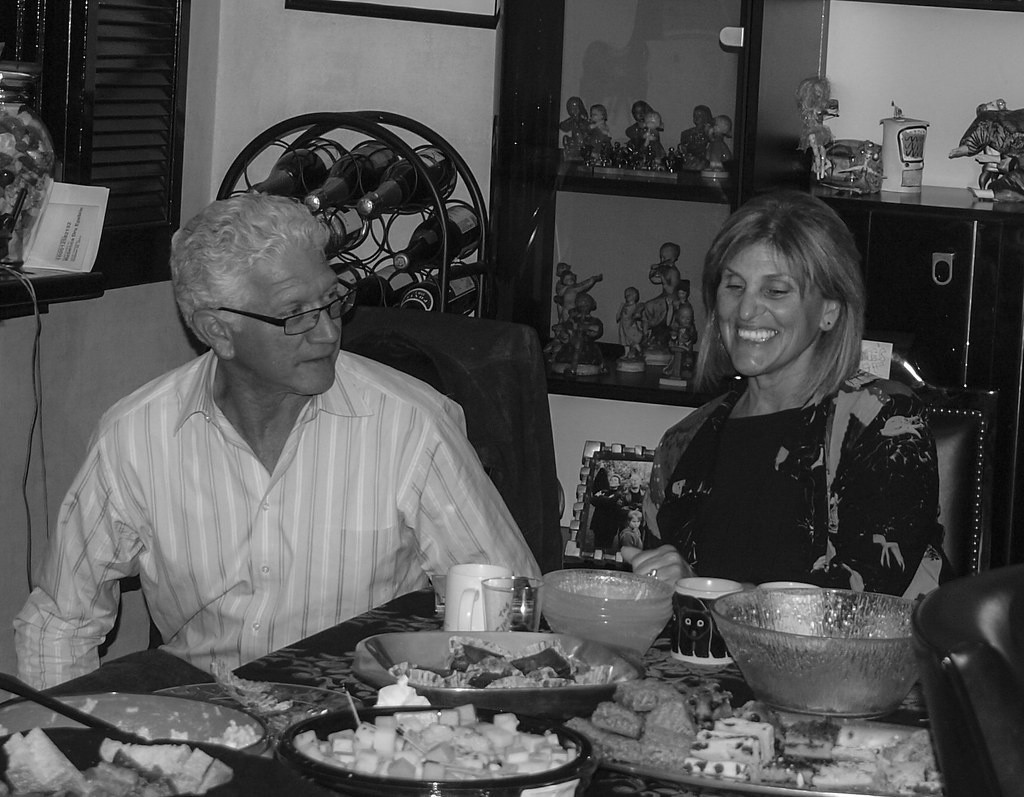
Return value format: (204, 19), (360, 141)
(354, 633), (637, 729)
(599, 706), (945, 797)
(150, 681), (365, 761)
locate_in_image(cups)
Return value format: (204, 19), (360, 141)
(880, 118), (929, 193)
(441, 563), (514, 632)
(431, 575), (447, 613)
(481, 575), (545, 634)
(671, 577), (744, 666)
(756, 581), (818, 588)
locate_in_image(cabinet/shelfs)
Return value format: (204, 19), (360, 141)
(488, 0), (1024, 564)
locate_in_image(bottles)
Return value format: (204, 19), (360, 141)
(392, 206), (481, 270)
(246, 145), (457, 219)
(321, 262), (487, 319)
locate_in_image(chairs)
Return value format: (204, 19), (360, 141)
(910, 389), (1016, 585)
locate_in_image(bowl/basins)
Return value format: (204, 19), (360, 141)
(278, 705), (600, 797)
(709, 588), (925, 718)
(543, 569), (677, 658)
(1, 690), (268, 758)
(0, 726), (295, 797)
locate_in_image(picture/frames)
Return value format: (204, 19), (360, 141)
(285, 0), (500, 29)
(564, 440), (658, 562)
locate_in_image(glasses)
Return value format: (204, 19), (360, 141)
(211, 277), (359, 335)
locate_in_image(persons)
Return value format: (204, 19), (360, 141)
(614, 242), (698, 378)
(795, 74), (833, 179)
(589, 460), (648, 553)
(547, 263), (604, 369)
(14, 185), (543, 697)
(559, 96), (733, 172)
(618, 187), (940, 617)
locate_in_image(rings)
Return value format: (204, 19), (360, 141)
(647, 568), (658, 579)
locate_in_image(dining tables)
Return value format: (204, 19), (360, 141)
(0, 583), (942, 797)
